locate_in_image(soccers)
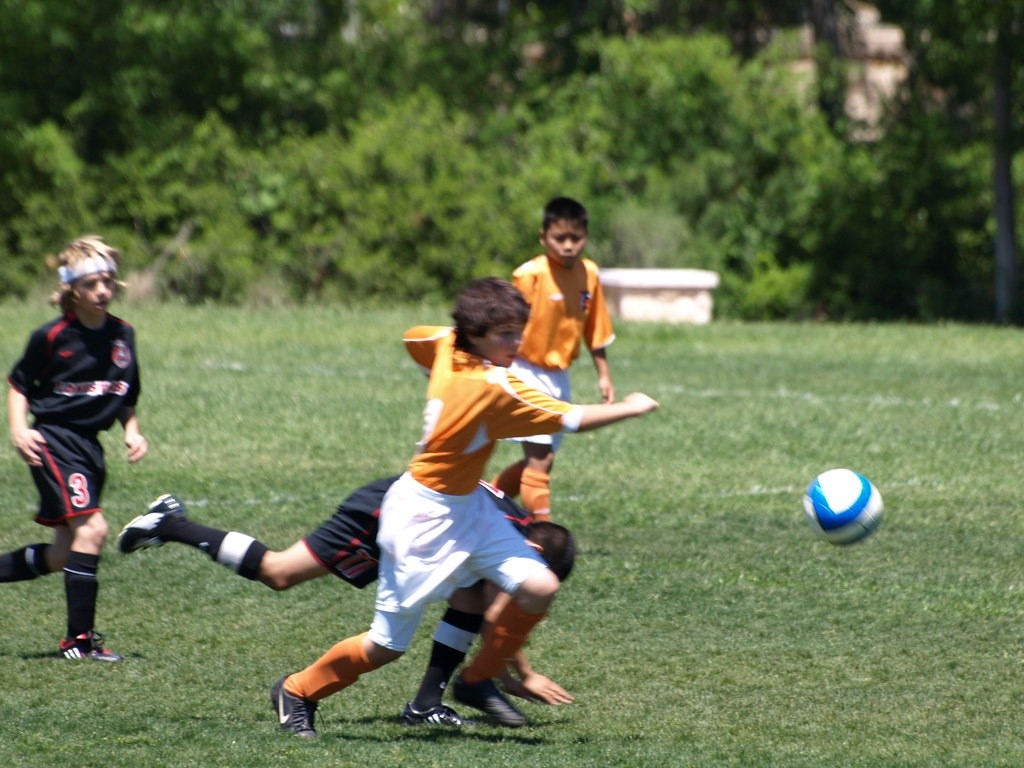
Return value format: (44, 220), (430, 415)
(803, 466), (885, 548)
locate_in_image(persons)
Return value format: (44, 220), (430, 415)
(0, 235), (148, 661)
(271, 277), (660, 743)
(489, 196), (617, 523)
(116, 473), (577, 726)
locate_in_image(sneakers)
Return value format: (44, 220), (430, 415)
(116, 494), (184, 555)
(453, 675), (525, 726)
(401, 701), (475, 728)
(59, 632), (125, 662)
(270, 674), (318, 741)
